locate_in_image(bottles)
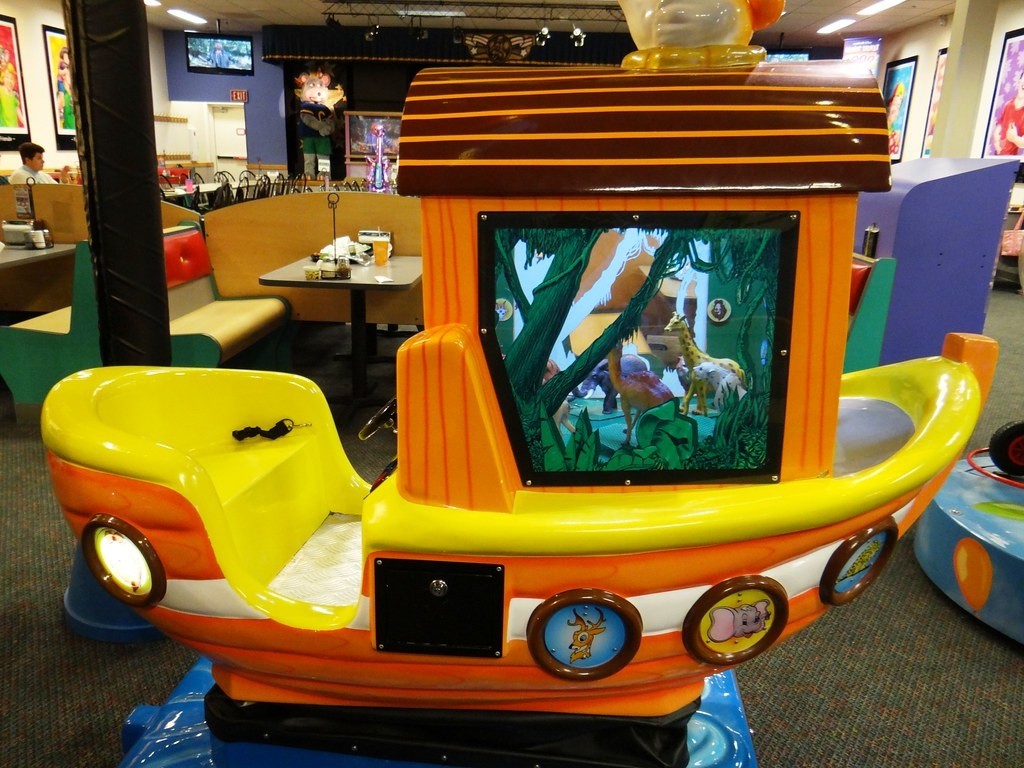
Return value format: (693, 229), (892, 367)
(337, 254), (350, 278)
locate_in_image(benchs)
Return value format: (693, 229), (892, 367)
(162, 221), (294, 367)
(1, 239), (103, 428)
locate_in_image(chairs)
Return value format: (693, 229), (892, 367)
(158, 171), (367, 214)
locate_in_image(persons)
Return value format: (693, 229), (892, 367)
(202, 42), (243, 68)
(10, 143), (71, 184)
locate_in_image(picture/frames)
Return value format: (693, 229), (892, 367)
(920, 46), (948, 158)
(883, 55), (919, 163)
(42, 24), (79, 150)
(980, 27), (1024, 182)
(0, 14), (32, 153)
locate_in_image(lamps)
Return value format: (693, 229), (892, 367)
(326, 14), (587, 48)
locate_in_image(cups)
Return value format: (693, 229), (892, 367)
(185, 179), (193, 192)
(267, 171), (279, 183)
(29, 231), (46, 249)
(373, 238), (389, 265)
(68, 172), (78, 184)
(304, 266), (320, 281)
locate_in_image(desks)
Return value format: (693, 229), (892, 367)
(160, 178), (291, 200)
(0, 244), (75, 270)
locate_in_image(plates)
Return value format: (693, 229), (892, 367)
(318, 243), (371, 253)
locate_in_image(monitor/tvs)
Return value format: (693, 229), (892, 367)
(766, 48), (813, 63)
(184, 31), (255, 77)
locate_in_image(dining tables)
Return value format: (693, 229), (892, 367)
(258, 253), (422, 409)
